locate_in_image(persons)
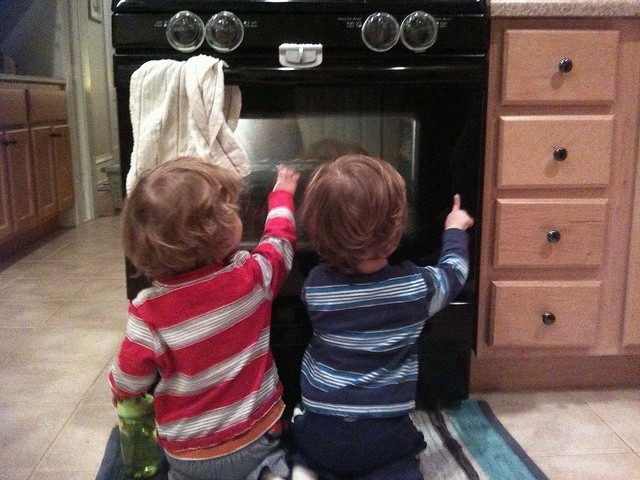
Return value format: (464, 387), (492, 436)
(285, 153), (475, 480)
(107, 154), (300, 480)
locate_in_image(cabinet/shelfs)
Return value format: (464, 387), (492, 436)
(0, 128), (34, 241)
(493, 197), (609, 272)
(485, 279), (604, 351)
(500, 26), (621, 105)
(30, 124), (75, 219)
(497, 114), (617, 192)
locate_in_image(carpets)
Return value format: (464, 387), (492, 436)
(95, 398), (549, 480)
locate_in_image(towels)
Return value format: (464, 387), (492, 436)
(27, 89), (69, 121)
(0, 89), (28, 127)
(126, 54), (252, 199)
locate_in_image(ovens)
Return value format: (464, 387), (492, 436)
(109, 3), (492, 418)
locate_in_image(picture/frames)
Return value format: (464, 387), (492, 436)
(88, 0), (104, 25)
